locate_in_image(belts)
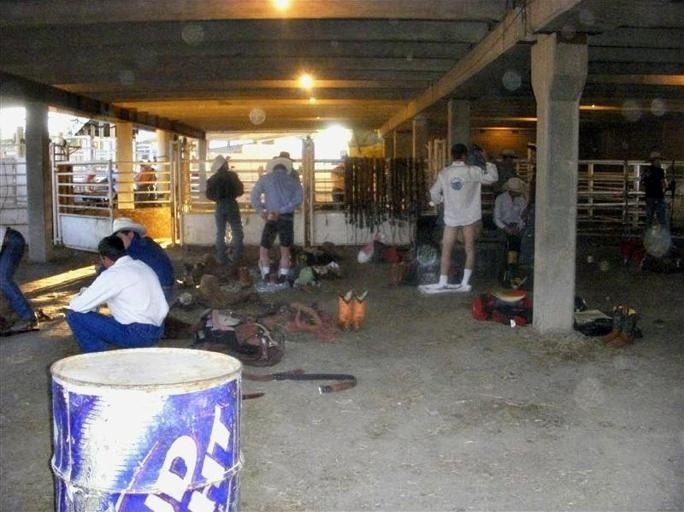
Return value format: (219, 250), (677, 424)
(272, 373), (356, 394)
(0, 227), (11, 260)
(241, 368), (304, 400)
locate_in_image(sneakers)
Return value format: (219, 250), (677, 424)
(257, 274), (271, 285)
(274, 274), (289, 284)
(10, 319), (40, 331)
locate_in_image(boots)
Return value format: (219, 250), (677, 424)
(353, 289), (369, 333)
(621, 308), (643, 344)
(601, 305), (623, 343)
(335, 288), (353, 333)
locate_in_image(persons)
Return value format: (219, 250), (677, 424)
(66, 235), (170, 353)
(205, 155), (244, 266)
(493, 150), (519, 197)
(134, 164), (157, 206)
(493, 178), (528, 287)
(0, 224), (38, 338)
(519, 143), (538, 267)
(84, 174), (97, 200)
(322, 186), (345, 210)
(330, 155), (349, 204)
(253, 157), (304, 285)
(95, 216), (173, 302)
(429, 144), (498, 291)
(641, 151), (669, 231)
(277, 152), (300, 183)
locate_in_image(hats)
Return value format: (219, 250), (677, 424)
(108, 217), (144, 237)
(273, 152), (296, 162)
(265, 156), (292, 176)
(499, 149), (518, 159)
(645, 151), (667, 163)
(212, 155), (229, 170)
(503, 177), (527, 194)
